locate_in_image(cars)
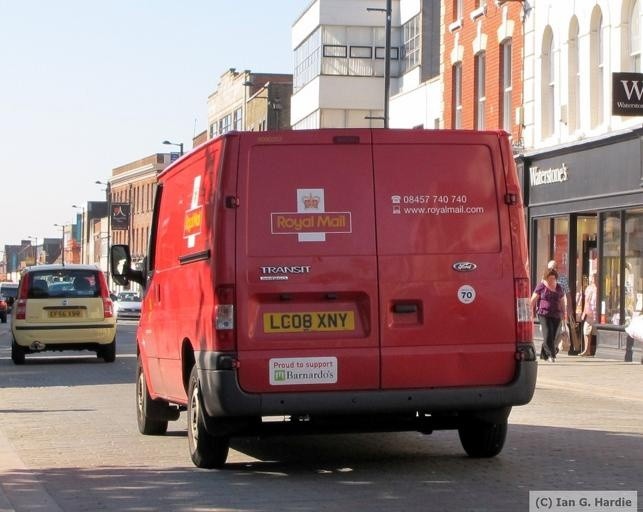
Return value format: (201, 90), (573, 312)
(110, 291), (142, 323)
(8, 266), (117, 363)
(0, 295), (7, 322)
(47, 282), (73, 290)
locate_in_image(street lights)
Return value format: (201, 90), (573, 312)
(27, 234), (37, 265)
(0, 250), (12, 281)
(52, 223), (65, 265)
(158, 137), (185, 160)
(241, 80), (281, 132)
(71, 204), (84, 265)
(93, 178), (113, 288)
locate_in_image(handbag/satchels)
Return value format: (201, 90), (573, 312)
(552, 319), (570, 352)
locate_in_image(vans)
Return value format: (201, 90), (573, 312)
(109, 128), (538, 470)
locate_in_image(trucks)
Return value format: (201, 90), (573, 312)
(0, 281), (22, 312)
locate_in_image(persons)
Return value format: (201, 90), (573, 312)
(616, 261), (633, 317)
(529, 260), (599, 363)
(113, 205), (126, 217)
(77, 278), (91, 291)
